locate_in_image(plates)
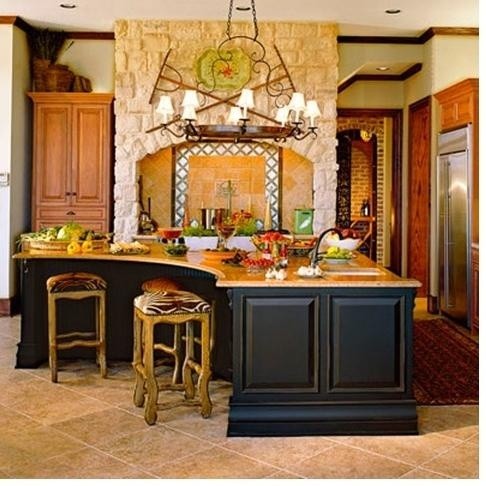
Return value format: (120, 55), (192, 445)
(292, 271), (326, 279)
(316, 253), (355, 264)
(109, 247), (152, 255)
(198, 249), (252, 261)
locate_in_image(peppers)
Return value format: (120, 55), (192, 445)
(67, 242), (80, 253)
(82, 241), (92, 253)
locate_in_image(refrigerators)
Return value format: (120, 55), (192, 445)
(435, 123), (473, 332)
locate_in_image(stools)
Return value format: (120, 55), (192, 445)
(46, 272), (108, 382)
(132, 290), (213, 425)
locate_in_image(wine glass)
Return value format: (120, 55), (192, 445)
(216, 224), (238, 253)
(157, 226), (184, 249)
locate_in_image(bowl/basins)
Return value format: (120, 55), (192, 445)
(326, 237), (361, 252)
(160, 244), (191, 258)
(287, 246), (315, 257)
(251, 238), (290, 254)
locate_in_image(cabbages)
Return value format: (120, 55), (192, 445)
(58, 224), (85, 240)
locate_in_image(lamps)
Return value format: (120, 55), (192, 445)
(154, 88), (320, 142)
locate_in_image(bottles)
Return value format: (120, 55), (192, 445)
(360, 200), (370, 217)
(139, 210), (155, 234)
(271, 243), (282, 264)
(262, 240), (271, 261)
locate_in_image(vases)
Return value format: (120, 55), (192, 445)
(32, 58), (74, 91)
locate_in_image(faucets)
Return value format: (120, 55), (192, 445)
(313, 228), (343, 259)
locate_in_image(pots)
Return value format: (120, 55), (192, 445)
(198, 207), (227, 231)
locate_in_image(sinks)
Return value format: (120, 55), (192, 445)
(324, 268), (384, 275)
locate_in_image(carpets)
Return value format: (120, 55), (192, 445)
(412, 318), (479, 406)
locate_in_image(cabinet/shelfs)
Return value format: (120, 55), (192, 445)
(24, 90), (115, 234)
